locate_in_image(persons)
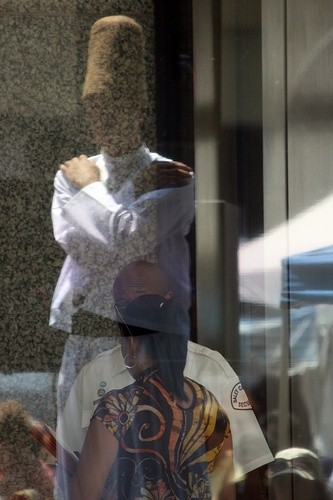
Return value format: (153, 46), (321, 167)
(68, 294), (237, 500)
(251, 375), (333, 500)
(0, 400), (54, 500)
(50, 72), (193, 499)
(55, 262), (275, 500)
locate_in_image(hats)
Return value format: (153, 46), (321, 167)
(81, 16), (151, 104)
